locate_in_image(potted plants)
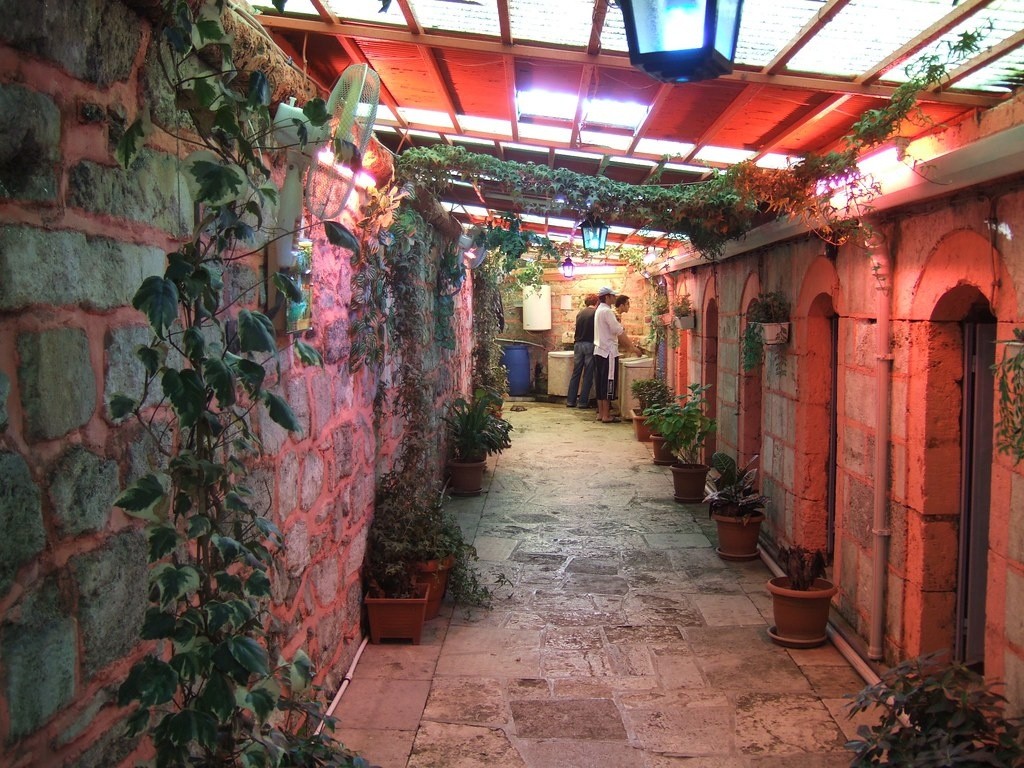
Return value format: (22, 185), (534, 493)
(736, 291), (791, 376)
(988, 328), (1024, 468)
(630, 378), (676, 442)
(642, 383), (719, 503)
(641, 294), (694, 351)
(702, 453), (772, 561)
(767, 540), (837, 648)
(365, 374), (514, 645)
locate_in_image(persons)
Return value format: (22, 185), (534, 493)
(594, 287), (642, 423)
(566, 294), (630, 409)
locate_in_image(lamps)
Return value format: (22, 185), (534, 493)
(620, 0), (742, 82)
(562, 255), (574, 278)
(577, 214), (608, 252)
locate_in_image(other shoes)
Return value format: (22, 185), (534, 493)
(579, 404), (593, 409)
(609, 410), (621, 416)
(566, 404), (577, 407)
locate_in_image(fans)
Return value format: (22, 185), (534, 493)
(268, 63), (380, 331)
(453, 223), (488, 287)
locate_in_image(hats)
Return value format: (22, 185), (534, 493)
(597, 286), (619, 296)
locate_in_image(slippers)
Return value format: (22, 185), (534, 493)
(602, 416), (621, 423)
(596, 417), (602, 421)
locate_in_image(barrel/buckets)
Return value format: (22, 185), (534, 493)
(499, 344), (530, 396)
